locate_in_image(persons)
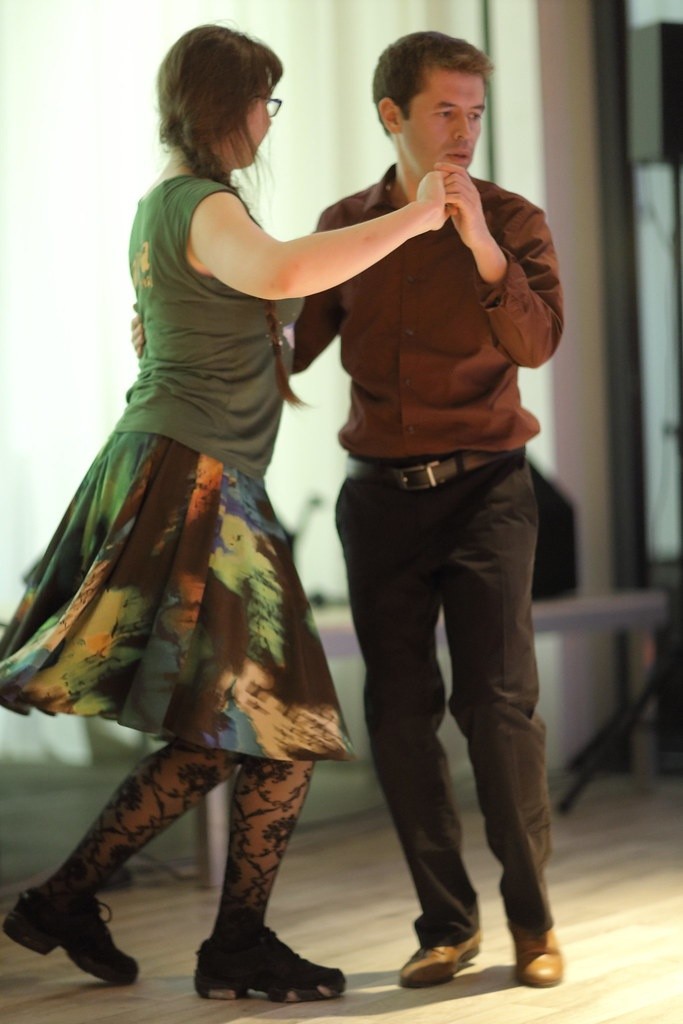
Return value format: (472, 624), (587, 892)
(0, 21), (453, 1006)
(127, 29), (568, 988)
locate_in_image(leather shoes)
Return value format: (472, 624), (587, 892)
(507, 920), (564, 990)
(398, 921), (485, 988)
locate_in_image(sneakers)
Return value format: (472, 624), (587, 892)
(194, 926), (347, 1004)
(2, 887), (140, 986)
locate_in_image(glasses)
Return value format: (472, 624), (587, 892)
(256, 92), (282, 119)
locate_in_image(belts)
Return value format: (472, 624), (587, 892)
(344, 445), (526, 493)
